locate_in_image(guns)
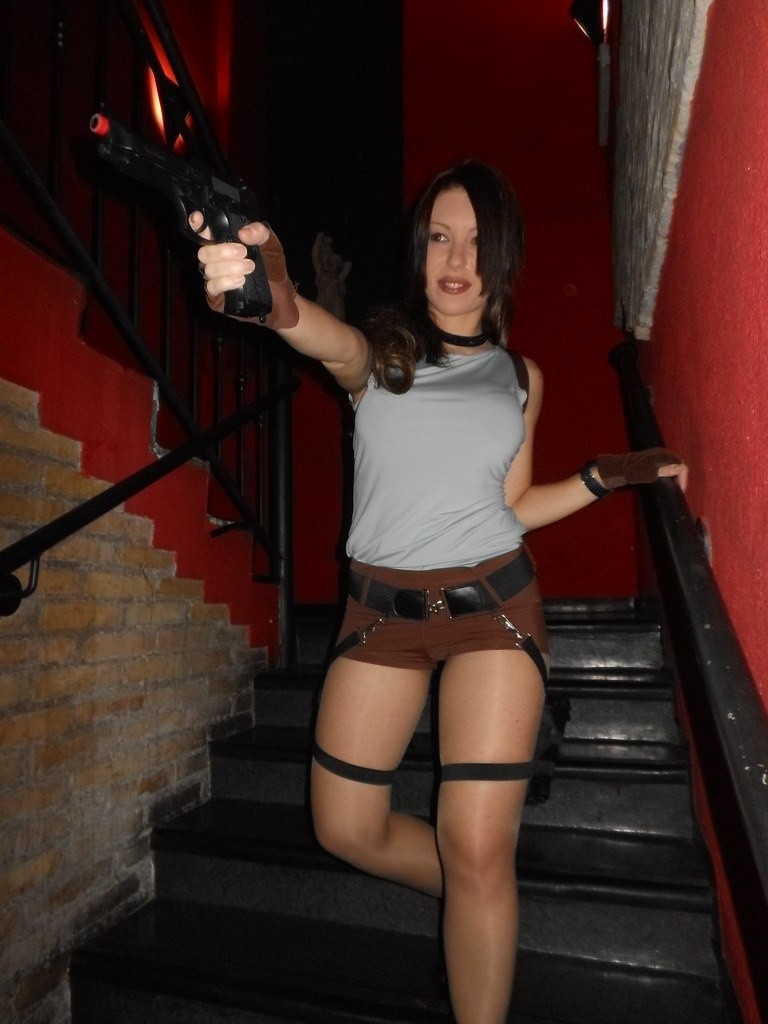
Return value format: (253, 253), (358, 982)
(89, 113), (277, 327)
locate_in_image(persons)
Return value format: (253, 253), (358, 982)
(188, 160), (689, 1024)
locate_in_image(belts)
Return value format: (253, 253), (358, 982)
(347, 548), (534, 622)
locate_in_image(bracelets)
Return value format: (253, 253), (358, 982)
(579, 460), (609, 498)
